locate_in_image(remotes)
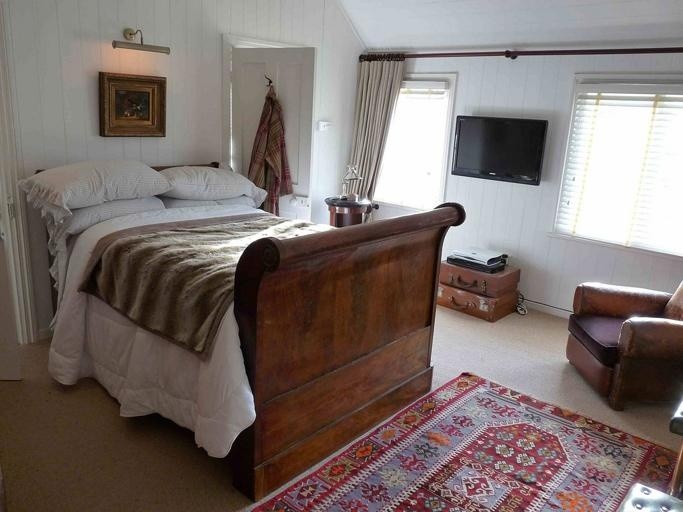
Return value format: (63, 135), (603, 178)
(340, 195), (347, 200)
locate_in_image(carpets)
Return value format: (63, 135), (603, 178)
(248, 370), (682, 512)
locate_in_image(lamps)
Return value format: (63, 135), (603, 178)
(111, 28), (171, 56)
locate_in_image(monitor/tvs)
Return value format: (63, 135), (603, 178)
(451, 115), (548, 186)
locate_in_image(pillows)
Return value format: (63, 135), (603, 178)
(17, 161), (267, 236)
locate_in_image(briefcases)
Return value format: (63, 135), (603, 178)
(440, 259), (521, 298)
(437, 283), (519, 322)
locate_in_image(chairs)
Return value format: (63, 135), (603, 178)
(565, 280), (683, 411)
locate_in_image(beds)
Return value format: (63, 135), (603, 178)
(31, 162), (465, 502)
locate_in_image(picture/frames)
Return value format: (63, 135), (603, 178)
(97, 71), (166, 138)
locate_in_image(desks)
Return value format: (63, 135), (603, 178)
(323, 196), (371, 228)
